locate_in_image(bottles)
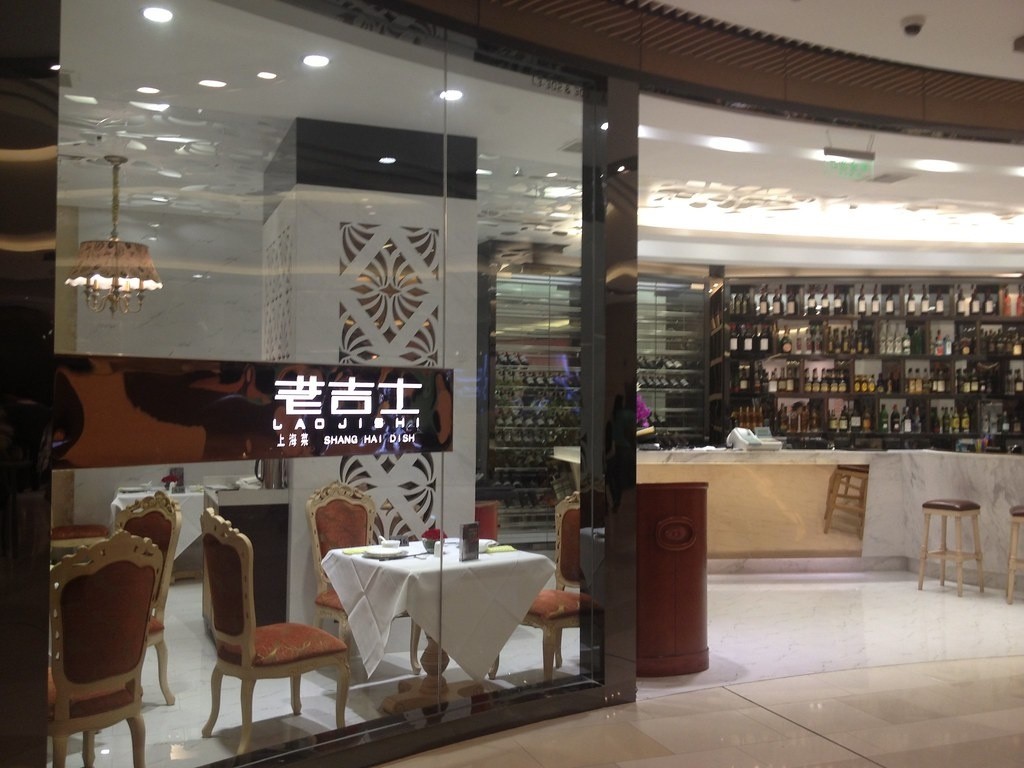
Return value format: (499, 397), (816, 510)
(655, 432), (689, 448)
(495, 389), (581, 407)
(858, 285), (894, 315)
(647, 413), (669, 428)
(638, 355), (682, 370)
(907, 286), (944, 316)
(169, 478), (175, 494)
(489, 450), (546, 467)
(496, 371), (580, 387)
(491, 471), (553, 488)
(956, 289), (993, 316)
(1003, 288), (1024, 317)
(496, 352), (528, 369)
(638, 376), (690, 389)
(730, 323), (1024, 454)
(495, 429), (583, 447)
(496, 492), (534, 509)
(496, 409), (582, 427)
(735, 289), (797, 316)
(807, 288), (841, 315)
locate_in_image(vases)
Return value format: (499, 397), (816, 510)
(164, 481), (177, 488)
(422, 538), (445, 553)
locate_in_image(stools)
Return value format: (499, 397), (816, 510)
(1005, 505), (1024, 604)
(917, 498), (986, 597)
(824, 465), (870, 541)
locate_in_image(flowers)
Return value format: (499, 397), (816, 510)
(161, 475), (178, 482)
(421, 529), (447, 539)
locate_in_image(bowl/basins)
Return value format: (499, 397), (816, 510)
(140, 483), (152, 490)
(479, 543), (488, 552)
(422, 539), (445, 553)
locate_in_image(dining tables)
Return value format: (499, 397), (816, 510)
(110, 485), (204, 585)
(321, 536), (556, 717)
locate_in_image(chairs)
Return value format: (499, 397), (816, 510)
(199, 506), (350, 756)
(485, 490), (579, 681)
(46, 530), (164, 768)
(112, 489), (182, 706)
(306, 479), (422, 676)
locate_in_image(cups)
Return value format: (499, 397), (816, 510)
(382, 540), (400, 548)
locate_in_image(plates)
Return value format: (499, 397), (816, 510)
(362, 547), (423, 556)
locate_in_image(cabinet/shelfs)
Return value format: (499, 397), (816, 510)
(710, 275), (1024, 455)
(479, 251), (722, 545)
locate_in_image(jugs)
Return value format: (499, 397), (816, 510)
(255, 442), (282, 489)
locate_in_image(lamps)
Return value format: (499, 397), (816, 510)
(64, 154), (164, 315)
